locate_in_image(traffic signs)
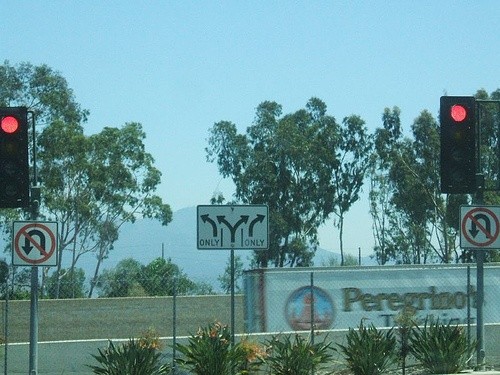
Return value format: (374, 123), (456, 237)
(197, 205), (270, 252)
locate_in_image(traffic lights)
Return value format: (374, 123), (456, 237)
(1, 106), (30, 209)
(440, 96), (477, 194)
